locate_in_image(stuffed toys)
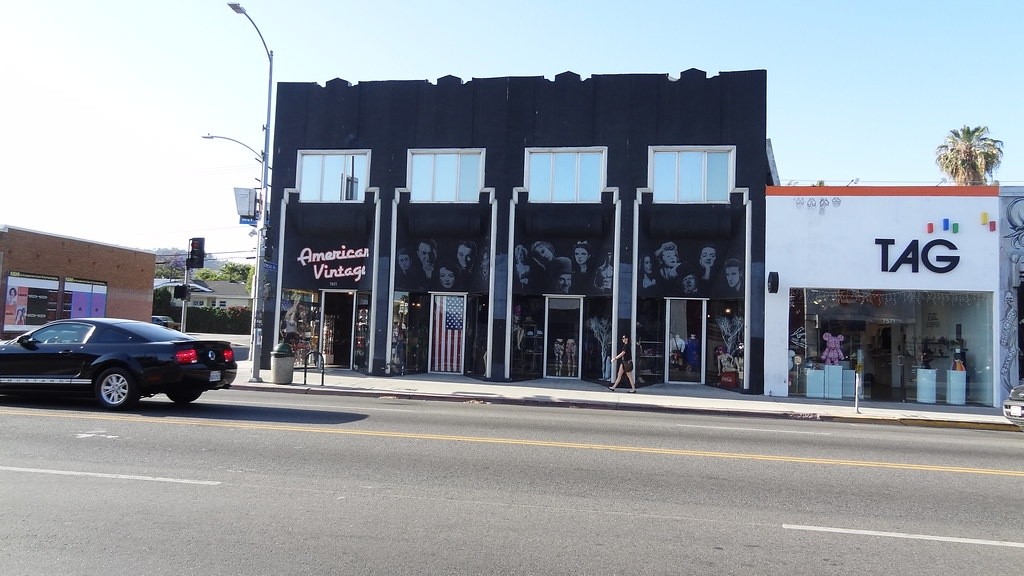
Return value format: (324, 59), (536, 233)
(821, 332), (844, 366)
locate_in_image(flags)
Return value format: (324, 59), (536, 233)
(434, 295), (464, 373)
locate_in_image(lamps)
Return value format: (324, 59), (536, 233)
(846, 177), (859, 188)
(936, 177), (948, 188)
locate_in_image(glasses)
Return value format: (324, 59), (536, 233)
(719, 349), (722, 351)
(622, 338), (626, 339)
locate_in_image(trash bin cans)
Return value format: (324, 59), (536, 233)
(269, 343), (295, 384)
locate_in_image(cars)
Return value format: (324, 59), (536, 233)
(0, 317), (239, 411)
(150, 316), (181, 331)
(1002, 384), (1024, 427)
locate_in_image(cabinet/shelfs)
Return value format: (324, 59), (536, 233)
(900, 335), (963, 405)
(640, 340), (663, 376)
(513, 326), (543, 371)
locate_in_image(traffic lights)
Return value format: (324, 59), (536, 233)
(189, 237), (205, 268)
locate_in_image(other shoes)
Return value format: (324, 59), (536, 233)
(607, 386), (614, 391)
(628, 390), (636, 393)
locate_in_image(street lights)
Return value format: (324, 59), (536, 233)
(199, 134), (267, 383)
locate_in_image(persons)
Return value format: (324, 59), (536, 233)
(512, 305), (524, 350)
(607, 334), (636, 393)
(396, 239), (490, 289)
(553, 338), (577, 377)
(638, 242), (745, 296)
(514, 241), (613, 295)
(670, 334), (701, 366)
(483, 351), (487, 377)
(717, 346), (737, 376)
(15, 307), (26, 325)
(7, 288), (18, 305)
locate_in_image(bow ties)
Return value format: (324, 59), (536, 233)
(423, 265), (434, 271)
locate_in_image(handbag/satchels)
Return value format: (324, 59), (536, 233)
(623, 361), (633, 372)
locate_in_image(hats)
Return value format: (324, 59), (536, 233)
(549, 257), (576, 274)
(676, 262), (706, 279)
